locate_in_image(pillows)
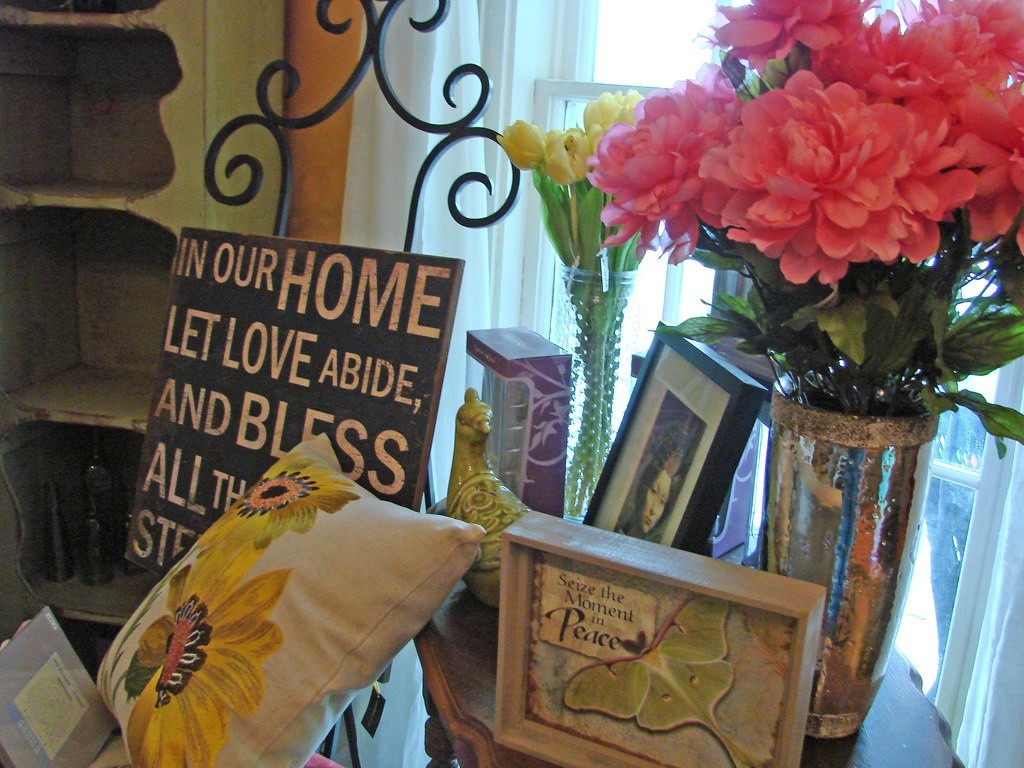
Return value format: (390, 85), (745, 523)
(96, 431), (484, 766)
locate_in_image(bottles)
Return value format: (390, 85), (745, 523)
(44, 427), (148, 586)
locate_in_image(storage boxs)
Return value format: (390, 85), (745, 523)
(469, 324), (574, 519)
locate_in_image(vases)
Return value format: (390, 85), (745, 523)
(563, 273), (637, 519)
(763, 391), (943, 739)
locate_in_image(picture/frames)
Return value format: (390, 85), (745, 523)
(583, 322), (764, 547)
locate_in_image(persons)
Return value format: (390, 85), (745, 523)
(613, 459), (674, 539)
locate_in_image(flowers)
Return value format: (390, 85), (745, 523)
(496, 90), (664, 272)
(585, 0), (1024, 458)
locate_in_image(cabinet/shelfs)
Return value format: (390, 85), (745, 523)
(1, 0), (283, 628)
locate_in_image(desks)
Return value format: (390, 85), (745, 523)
(414, 491), (964, 766)
